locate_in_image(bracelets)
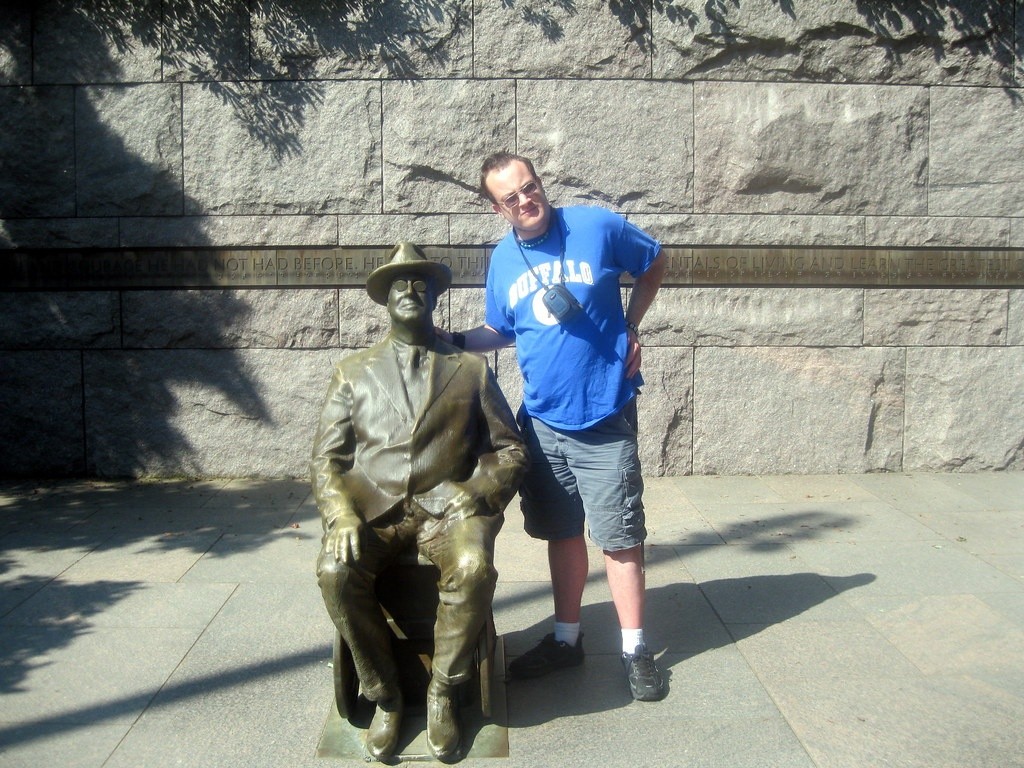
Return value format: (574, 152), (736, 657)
(625, 320), (639, 336)
(451, 332), (465, 350)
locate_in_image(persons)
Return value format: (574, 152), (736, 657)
(433, 152), (667, 702)
(311, 242), (529, 760)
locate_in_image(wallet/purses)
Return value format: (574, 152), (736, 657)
(542, 285), (583, 329)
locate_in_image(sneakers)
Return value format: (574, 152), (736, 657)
(508, 631), (586, 678)
(621, 643), (664, 701)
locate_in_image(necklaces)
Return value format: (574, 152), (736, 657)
(516, 219), (552, 248)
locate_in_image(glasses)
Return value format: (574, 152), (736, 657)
(497, 178), (538, 209)
